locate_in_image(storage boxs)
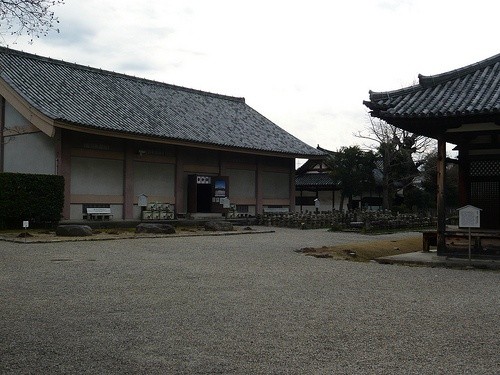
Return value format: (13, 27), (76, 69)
(141, 203), (174, 220)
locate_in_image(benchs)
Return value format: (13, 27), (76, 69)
(92, 213), (115, 221)
(413, 229), (500, 252)
